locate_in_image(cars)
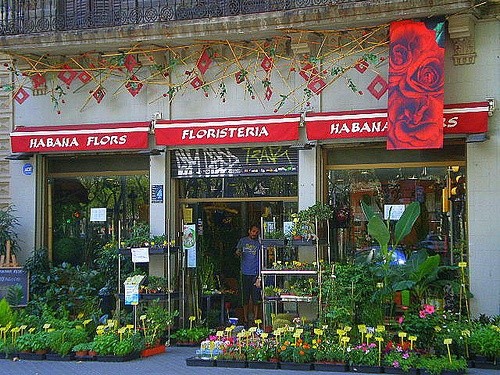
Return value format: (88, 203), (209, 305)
(350, 231), (459, 268)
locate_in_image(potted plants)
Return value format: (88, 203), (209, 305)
(433, 309), (500, 370)
(416, 352), (469, 375)
(0, 222), (181, 361)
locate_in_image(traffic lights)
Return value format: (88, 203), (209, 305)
(447, 165), (465, 200)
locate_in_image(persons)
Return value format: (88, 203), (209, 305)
(236, 225), (261, 324)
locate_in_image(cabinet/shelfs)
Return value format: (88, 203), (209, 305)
(116, 219), (185, 346)
(257, 216), (331, 327)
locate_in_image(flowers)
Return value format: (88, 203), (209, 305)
(194, 215), (451, 374)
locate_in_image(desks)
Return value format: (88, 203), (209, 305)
(200, 292), (226, 325)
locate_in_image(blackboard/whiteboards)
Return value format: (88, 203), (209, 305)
(0, 267), (29, 306)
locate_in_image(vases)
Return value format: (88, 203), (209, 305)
(312, 361), (348, 371)
(247, 360), (278, 369)
(261, 238), (289, 247)
(281, 292), (290, 296)
(293, 235), (301, 240)
(216, 359), (248, 369)
(290, 293), (296, 296)
(185, 356), (215, 366)
(264, 296), (282, 301)
(349, 364), (382, 373)
(279, 361), (313, 370)
(383, 365), (417, 375)
(291, 238), (317, 246)
